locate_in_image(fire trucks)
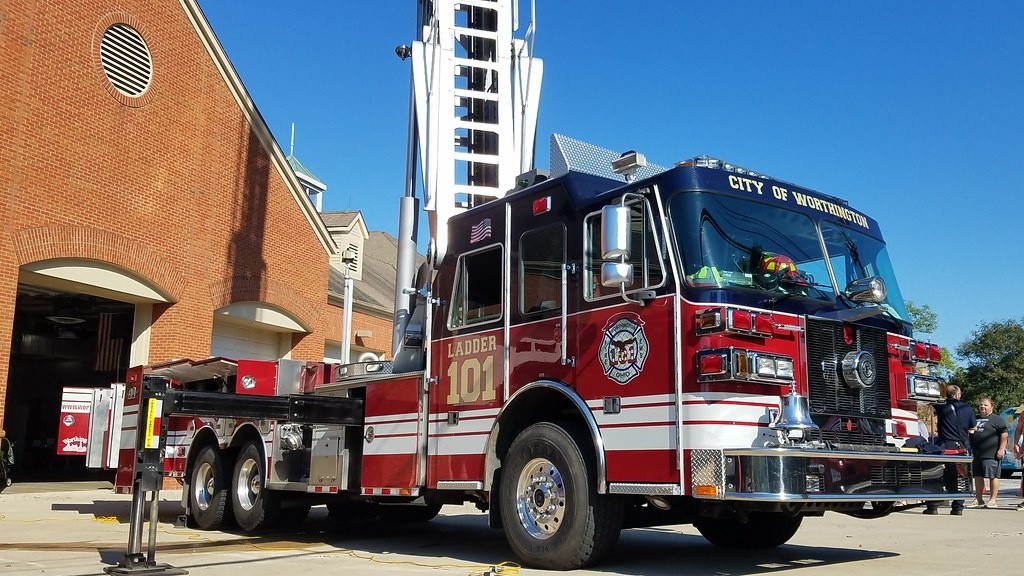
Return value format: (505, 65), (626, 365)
(56, 0), (976, 572)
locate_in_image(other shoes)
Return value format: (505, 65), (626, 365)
(950, 509), (962, 515)
(966, 497), (985, 508)
(984, 501), (997, 508)
(922, 508), (938, 515)
(1017, 501), (1024, 511)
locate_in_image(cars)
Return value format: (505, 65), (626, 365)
(998, 406), (1023, 478)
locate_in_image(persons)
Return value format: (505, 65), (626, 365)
(922, 385), (978, 516)
(969, 394), (1009, 509)
(1013, 396), (1024, 508)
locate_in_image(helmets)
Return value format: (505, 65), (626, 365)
(759, 254), (805, 284)
